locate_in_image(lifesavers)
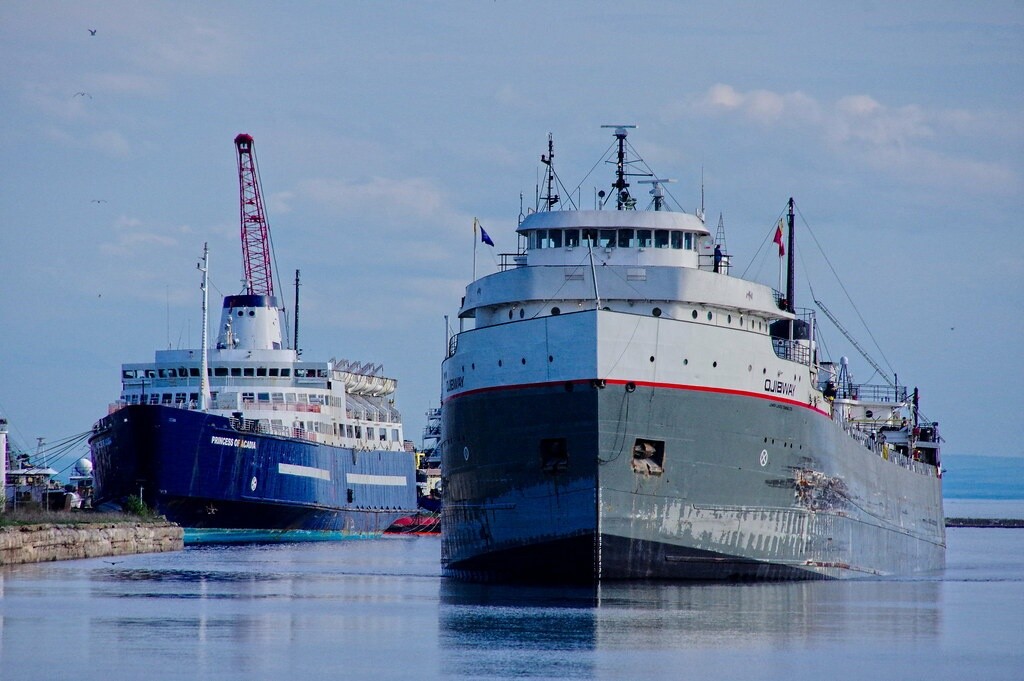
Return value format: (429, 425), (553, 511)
(883, 446), (889, 459)
(914, 449), (922, 461)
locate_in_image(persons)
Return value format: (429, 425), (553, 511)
(713, 245), (723, 273)
(900, 417), (909, 430)
(827, 380), (836, 410)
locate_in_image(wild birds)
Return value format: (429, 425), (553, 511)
(89, 29), (96, 35)
(91, 199), (106, 204)
(103, 561), (123, 565)
(74, 91), (92, 98)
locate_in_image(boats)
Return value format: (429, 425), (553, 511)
(383, 399), (441, 536)
(88, 133), (420, 542)
(5, 454), (67, 508)
(440, 124), (949, 582)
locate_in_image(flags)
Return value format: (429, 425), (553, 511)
(772, 218), (785, 256)
(480, 225), (494, 247)
